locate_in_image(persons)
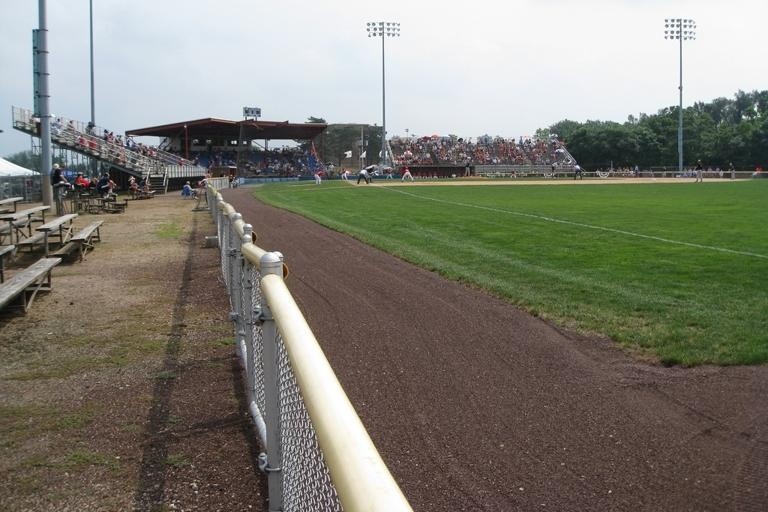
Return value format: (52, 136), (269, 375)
(661, 166), (667, 177)
(212, 146), (324, 185)
(29, 110), (212, 200)
(595, 164), (654, 176)
(395, 137), (573, 164)
(329, 162), (414, 184)
(510, 168), (517, 179)
(681, 162), (735, 177)
(694, 159), (703, 183)
(413, 170), (438, 178)
(752, 164), (763, 176)
(573, 165), (582, 180)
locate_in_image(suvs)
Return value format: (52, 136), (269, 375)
(3, 179), (40, 199)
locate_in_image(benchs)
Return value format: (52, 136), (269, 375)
(0, 184), (155, 318)
(389, 133), (571, 169)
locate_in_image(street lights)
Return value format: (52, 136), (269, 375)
(663, 16), (697, 171)
(364, 20), (401, 166)
